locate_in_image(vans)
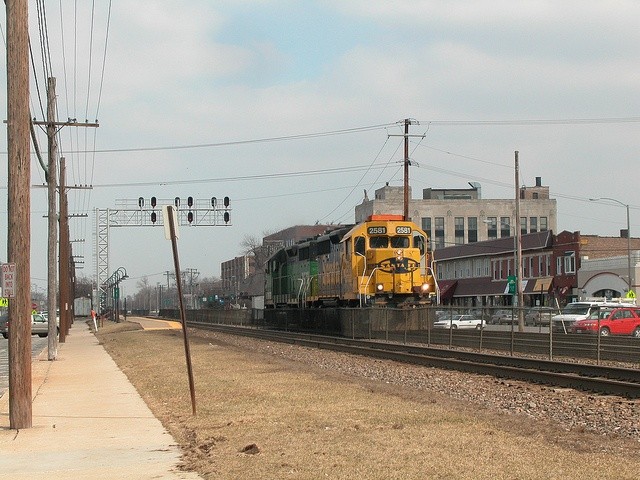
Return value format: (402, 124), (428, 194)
(553, 303), (618, 333)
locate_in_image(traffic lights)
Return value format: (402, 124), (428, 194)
(188, 212), (193, 223)
(225, 212), (229, 223)
(188, 197), (193, 206)
(224, 197), (229, 207)
(151, 213), (156, 224)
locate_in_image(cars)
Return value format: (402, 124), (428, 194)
(434, 315), (487, 331)
(525, 307), (557, 326)
(492, 310), (519, 325)
(41, 310), (60, 326)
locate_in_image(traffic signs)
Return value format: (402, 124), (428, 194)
(151, 197), (156, 207)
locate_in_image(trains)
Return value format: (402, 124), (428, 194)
(264, 214), (441, 338)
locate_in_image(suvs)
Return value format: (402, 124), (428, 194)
(0, 310), (60, 339)
(573, 308), (640, 337)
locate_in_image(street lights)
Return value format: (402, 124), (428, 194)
(482, 219), (519, 311)
(105, 275), (116, 322)
(117, 267), (129, 323)
(116, 270), (126, 323)
(231, 275), (237, 305)
(588, 197), (632, 291)
(113, 272), (122, 321)
